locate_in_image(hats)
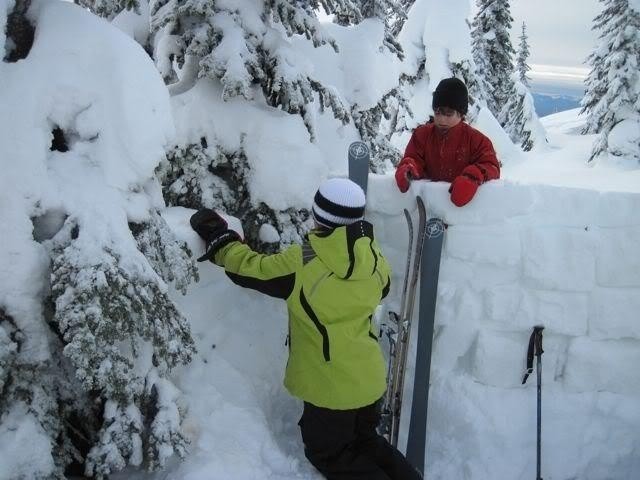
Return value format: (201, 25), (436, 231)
(312, 178), (366, 229)
(432, 78), (468, 115)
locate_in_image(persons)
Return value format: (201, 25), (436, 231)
(189, 179), (424, 480)
(396, 76), (501, 206)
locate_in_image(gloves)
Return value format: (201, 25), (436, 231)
(395, 157), (420, 191)
(448, 165), (484, 206)
(190, 208), (242, 262)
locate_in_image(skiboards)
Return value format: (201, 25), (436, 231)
(349, 142), (443, 480)
